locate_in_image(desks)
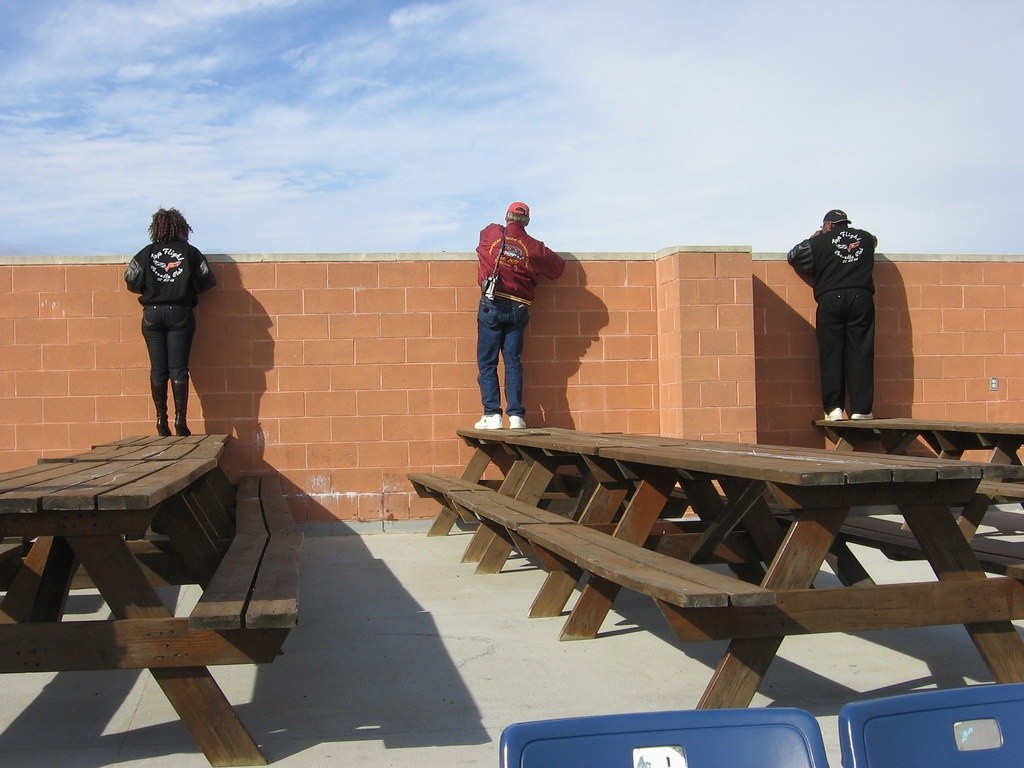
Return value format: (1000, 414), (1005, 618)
(425, 429), (624, 564)
(814, 418), (1024, 549)
(37, 445), (237, 559)
(475, 434), (820, 618)
(92, 433), (238, 520)
(0, 460), (270, 766)
(557, 445), (1024, 711)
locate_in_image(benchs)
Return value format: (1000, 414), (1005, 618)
(518, 524), (772, 640)
(0, 535), (37, 585)
(447, 490), (578, 555)
(235, 497), (300, 532)
(189, 531), (307, 656)
(406, 471), (495, 525)
(236, 474), (283, 500)
(667, 486), (1024, 583)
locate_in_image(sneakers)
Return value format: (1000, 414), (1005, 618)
(851, 411), (874, 420)
(824, 407), (843, 420)
(474, 413), (503, 429)
(509, 415), (526, 429)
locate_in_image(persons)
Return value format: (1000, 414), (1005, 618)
(124, 208), (217, 437)
(787, 209), (878, 422)
(474, 202), (565, 429)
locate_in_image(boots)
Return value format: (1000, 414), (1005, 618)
(151, 379), (171, 436)
(171, 378), (191, 436)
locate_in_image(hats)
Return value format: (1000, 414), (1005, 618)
(508, 201), (529, 217)
(823, 210), (852, 223)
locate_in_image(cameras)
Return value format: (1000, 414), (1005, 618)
(482, 275), (500, 302)
